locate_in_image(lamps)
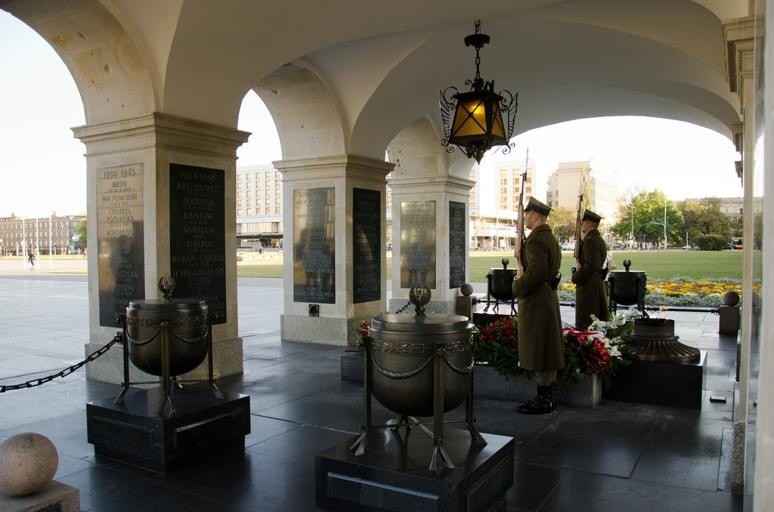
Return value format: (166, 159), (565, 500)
(439, 20), (519, 165)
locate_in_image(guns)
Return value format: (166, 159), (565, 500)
(513, 147), (528, 281)
(573, 175), (586, 273)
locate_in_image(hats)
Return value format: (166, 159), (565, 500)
(523, 195), (555, 216)
(580, 208), (605, 224)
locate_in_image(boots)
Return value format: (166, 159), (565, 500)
(514, 383), (560, 417)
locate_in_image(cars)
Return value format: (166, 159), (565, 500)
(562, 243), (575, 249)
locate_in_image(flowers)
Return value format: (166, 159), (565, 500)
(348, 314), (371, 342)
(472, 304), (649, 387)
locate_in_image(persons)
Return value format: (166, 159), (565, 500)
(83, 247), (88, 258)
(26, 243), (40, 266)
(571, 209), (608, 330)
(511, 196), (562, 415)
(625, 237), (661, 251)
